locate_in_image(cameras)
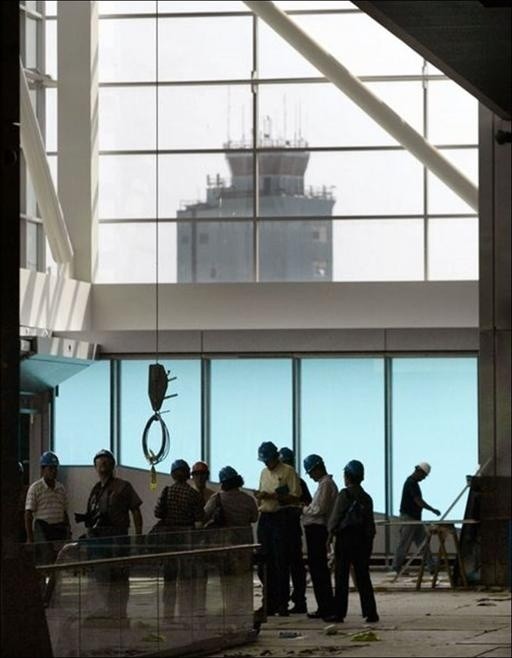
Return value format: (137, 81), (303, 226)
(74, 511), (98, 528)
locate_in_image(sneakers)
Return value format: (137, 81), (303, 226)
(255, 604), (379, 622)
(392, 566), (409, 575)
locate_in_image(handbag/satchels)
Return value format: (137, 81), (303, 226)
(203, 518), (240, 544)
(147, 521), (175, 553)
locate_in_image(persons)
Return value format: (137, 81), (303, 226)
(390, 462), (440, 577)
(156, 442), (340, 624)
(25, 451), (72, 607)
(74, 450), (144, 627)
(326, 458), (380, 623)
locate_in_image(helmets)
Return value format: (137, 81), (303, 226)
(419, 463), (430, 475)
(18, 461), (24, 473)
(258, 442), (295, 465)
(94, 449), (115, 466)
(171, 459), (238, 484)
(303, 455), (322, 474)
(344, 460), (364, 481)
(39, 452), (58, 467)
